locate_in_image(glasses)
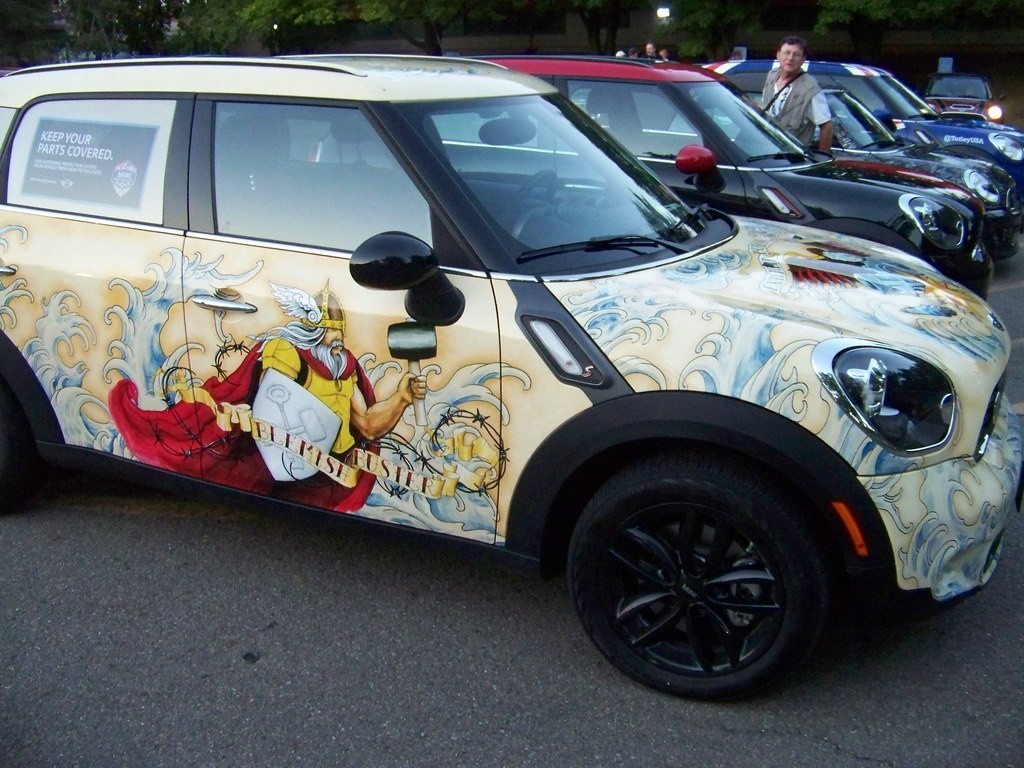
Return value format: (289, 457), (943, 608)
(779, 49), (803, 60)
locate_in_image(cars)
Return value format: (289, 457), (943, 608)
(0, 54), (1023, 707)
(307, 48), (1023, 310)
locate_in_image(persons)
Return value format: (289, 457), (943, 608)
(761, 35), (834, 152)
(616, 42), (669, 61)
